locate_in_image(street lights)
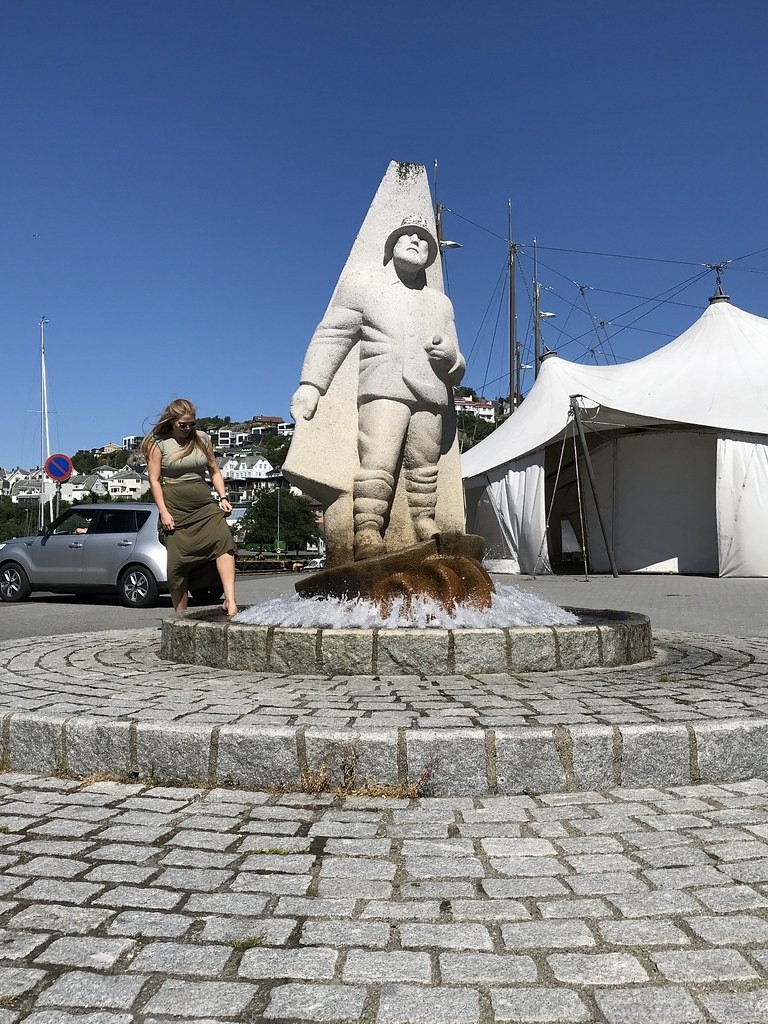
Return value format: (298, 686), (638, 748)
(534, 311), (556, 381)
(516, 364), (533, 410)
(275, 487), (280, 560)
(25, 509), (28, 537)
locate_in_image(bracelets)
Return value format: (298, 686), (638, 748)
(220, 497), (230, 502)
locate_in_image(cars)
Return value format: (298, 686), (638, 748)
(300, 559), (326, 573)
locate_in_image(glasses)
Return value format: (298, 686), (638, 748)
(176, 421), (196, 429)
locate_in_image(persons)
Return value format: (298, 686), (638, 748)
(289, 214), (467, 561)
(75, 527), (88, 533)
(141, 399), (239, 616)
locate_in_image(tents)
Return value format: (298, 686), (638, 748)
(461, 303), (768, 578)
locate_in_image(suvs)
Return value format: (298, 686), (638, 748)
(0, 502), (225, 609)
